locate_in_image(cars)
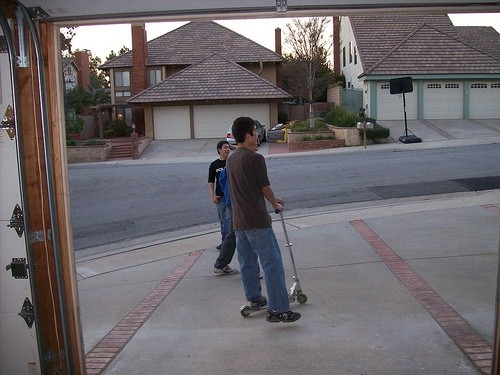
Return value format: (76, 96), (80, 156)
(227, 120), (267, 146)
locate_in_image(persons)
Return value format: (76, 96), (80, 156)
(208, 141), (263, 279)
(226, 116), (301, 323)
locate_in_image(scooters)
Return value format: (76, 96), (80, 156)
(240, 202), (307, 317)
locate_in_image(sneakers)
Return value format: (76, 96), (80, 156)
(214, 265), (238, 276)
(250, 296), (267, 311)
(267, 308), (301, 323)
(216, 244), (222, 249)
